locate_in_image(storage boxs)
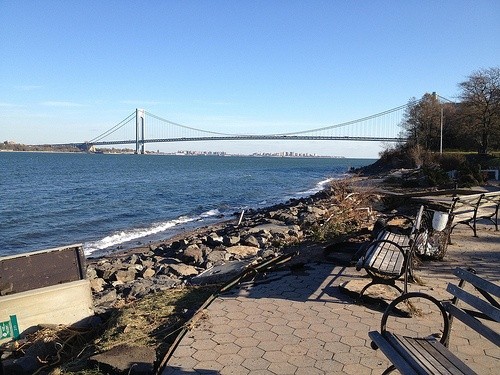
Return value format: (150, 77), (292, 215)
(0, 242), (95, 347)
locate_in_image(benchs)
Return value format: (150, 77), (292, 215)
(368, 265), (500, 375)
(356, 205), (425, 315)
(425, 190), (500, 244)
